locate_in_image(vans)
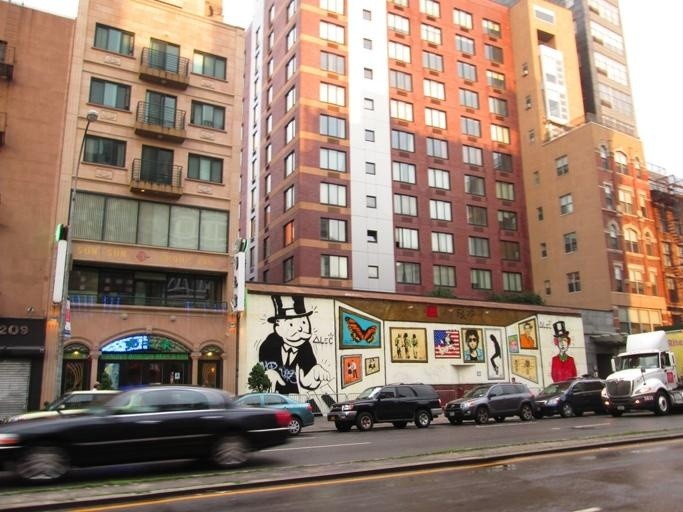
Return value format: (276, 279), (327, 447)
(534, 376), (605, 418)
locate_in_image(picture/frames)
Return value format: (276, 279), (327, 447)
(339, 305), (383, 350)
(434, 330), (462, 359)
(517, 318), (538, 350)
(341, 354), (362, 389)
(389, 326), (428, 363)
(365, 356), (381, 377)
(510, 353), (538, 385)
(461, 327), (485, 363)
(485, 328), (506, 380)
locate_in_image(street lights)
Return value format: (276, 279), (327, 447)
(52, 110), (101, 400)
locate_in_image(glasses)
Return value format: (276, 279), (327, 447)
(467, 338), (477, 342)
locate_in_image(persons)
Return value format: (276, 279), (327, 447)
(520, 323), (534, 347)
(395, 333), (419, 359)
(348, 360), (359, 383)
(464, 329), (483, 360)
(551, 332), (577, 382)
(92, 382), (101, 391)
(438, 333), (455, 355)
(490, 334), (501, 375)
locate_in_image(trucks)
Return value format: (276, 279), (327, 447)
(599, 328), (682, 415)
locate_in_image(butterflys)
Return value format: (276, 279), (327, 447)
(345, 316), (377, 343)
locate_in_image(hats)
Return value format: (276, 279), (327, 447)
(267, 294), (313, 323)
(553, 320), (569, 338)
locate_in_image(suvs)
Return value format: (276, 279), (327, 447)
(443, 381), (536, 425)
(327, 382), (442, 433)
